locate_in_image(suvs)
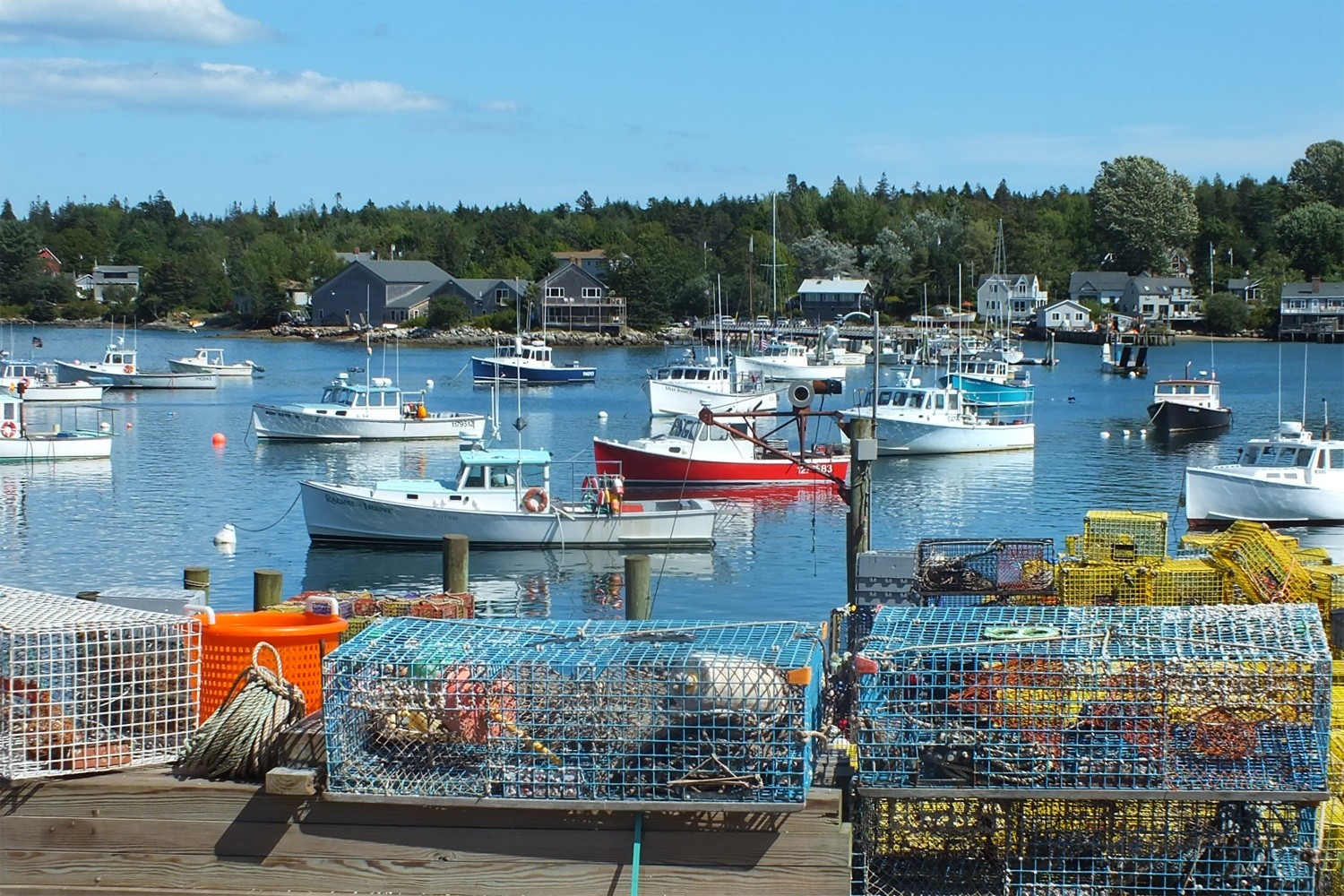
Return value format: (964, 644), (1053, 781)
(721, 315), (736, 326)
(776, 316), (790, 326)
(755, 315), (771, 327)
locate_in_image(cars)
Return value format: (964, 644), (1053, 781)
(793, 317), (807, 327)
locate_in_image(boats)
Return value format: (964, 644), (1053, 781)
(0, 315), (217, 400)
(834, 218), (1060, 456)
(168, 348), (266, 377)
(1100, 332), (1150, 374)
(642, 193), (867, 418)
(593, 402), (852, 525)
(472, 275), (597, 384)
(297, 337), (717, 550)
(252, 284), (486, 442)
(1147, 331), (1231, 432)
(1185, 331), (1344, 528)
(0, 377), (120, 463)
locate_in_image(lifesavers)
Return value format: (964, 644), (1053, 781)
(0, 421), (17, 438)
(523, 489), (548, 512)
(124, 365), (133, 373)
(19, 378), (29, 388)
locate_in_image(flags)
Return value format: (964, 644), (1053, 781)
(760, 330), (768, 350)
